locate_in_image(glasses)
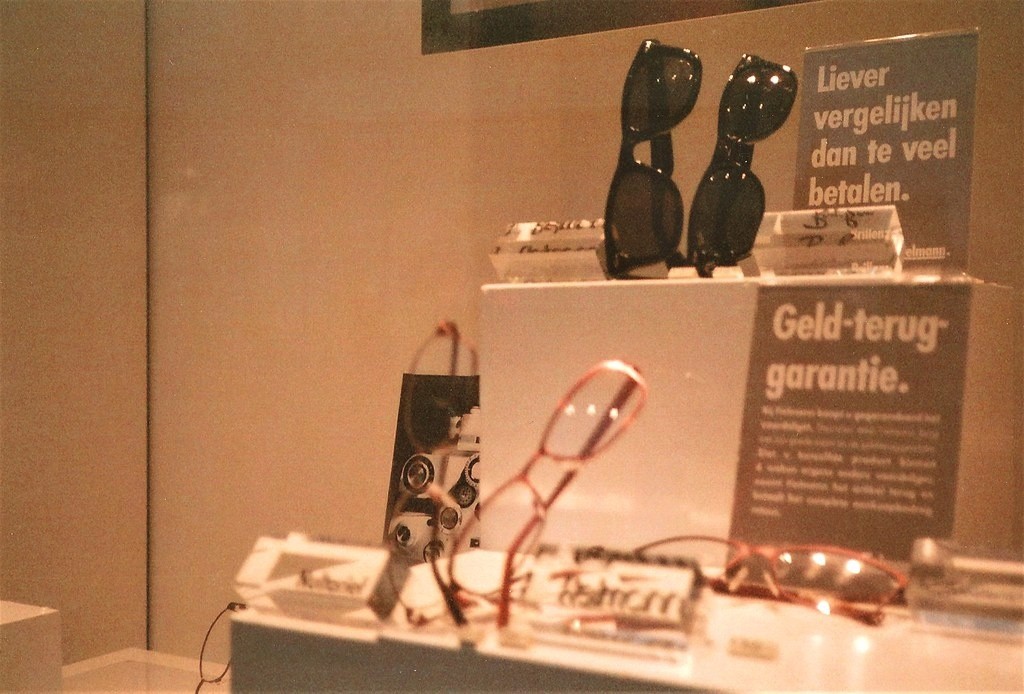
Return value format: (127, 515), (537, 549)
(446, 357), (650, 633)
(685, 53), (799, 280)
(383, 316), (484, 631)
(191, 601), (251, 694)
(634, 533), (907, 629)
(603, 36), (703, 278)
